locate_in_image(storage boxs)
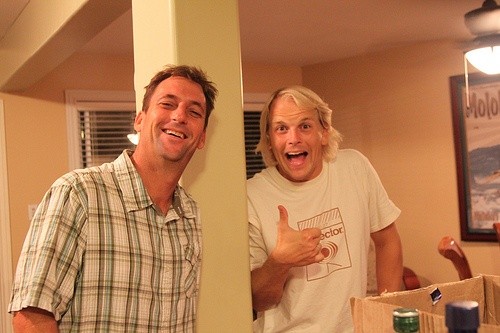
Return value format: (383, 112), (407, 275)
(350, 273), (500, 333)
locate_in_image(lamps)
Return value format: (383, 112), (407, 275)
(462, 0), (500, 76)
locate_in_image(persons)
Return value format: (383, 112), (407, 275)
(244, 84), (403, 333)
(7, 64), (219, 333)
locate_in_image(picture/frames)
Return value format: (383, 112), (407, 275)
(449, 71), (500, 243)
